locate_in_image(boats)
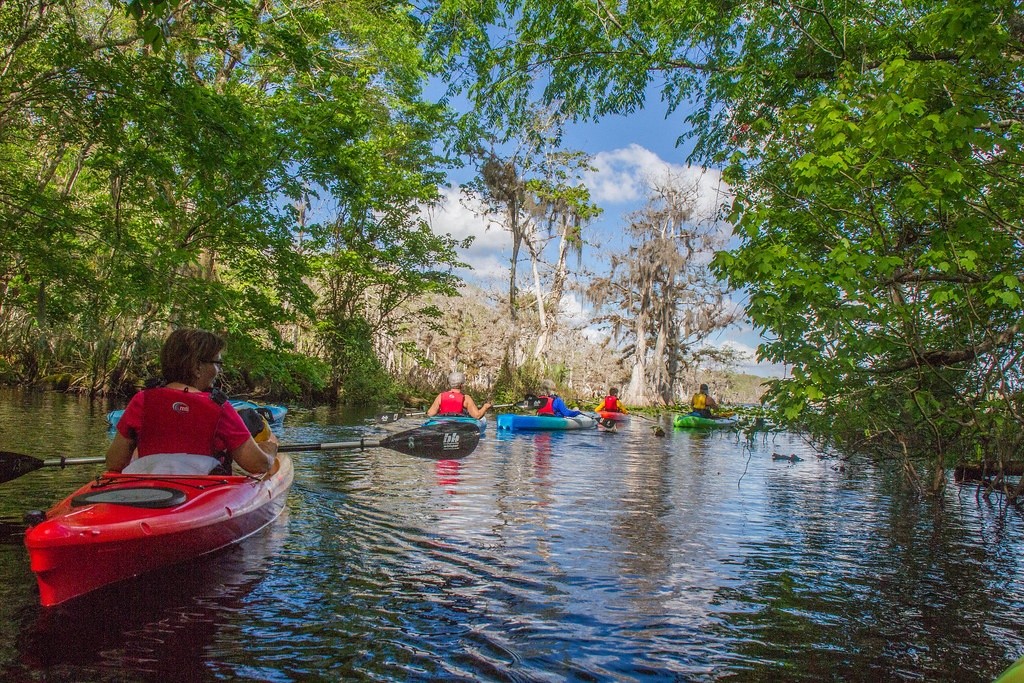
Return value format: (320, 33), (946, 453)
(674, 412), (738, 428)
(496, 412), (601, 429)
(421, 416), (487, 434)
(107, 399), (288, 427)
(597, 411), (631, 421)
(25, 436), (295, 609)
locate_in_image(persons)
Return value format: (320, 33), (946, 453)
(536, 380), (579, 417)
(104, 327), (279, 477)
(691, 383), (720, 420)
(595, 386), (630, 414)
(426, 371), (492, 420)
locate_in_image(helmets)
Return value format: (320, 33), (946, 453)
(541, 380), (555, 392)
(448, 372), (464, 387)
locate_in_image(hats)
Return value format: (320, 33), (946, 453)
(700, 384), (708, 393)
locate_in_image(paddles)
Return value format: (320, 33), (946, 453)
(373, 397), (549, 426)
(573, 398), (658, 423)
(0, 421), (484, 486)
(523, 393), (617, 429)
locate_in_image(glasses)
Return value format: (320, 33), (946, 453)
(199, 358), (224, 365)
